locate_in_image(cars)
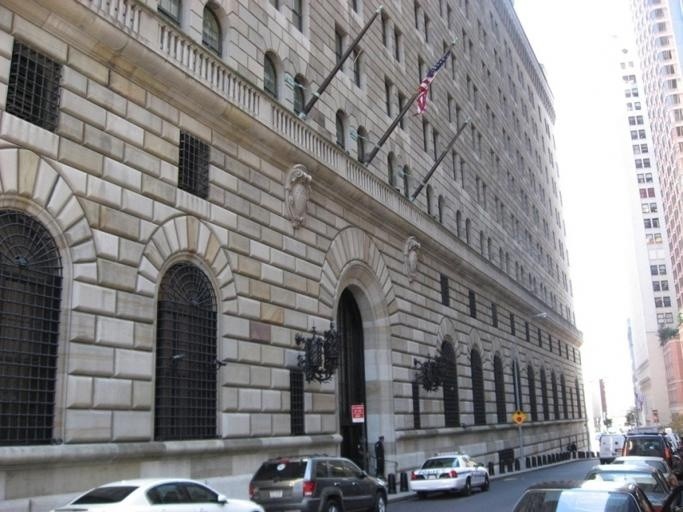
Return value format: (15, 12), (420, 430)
(508, 455), (683, 511)
(622, 425), (683, 475)
(409, 453), (490, 498)
(52, 476), (267, 512)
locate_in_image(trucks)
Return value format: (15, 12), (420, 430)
(595, 432), (626, 464)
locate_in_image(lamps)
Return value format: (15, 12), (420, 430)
(295, 318), (340, 385)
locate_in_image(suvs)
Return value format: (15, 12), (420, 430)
(248, 453), (389, 512)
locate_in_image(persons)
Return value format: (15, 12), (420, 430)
(375, 437), (384, 478)
(567, 442), (577, 452)
(354, 433), (369, 471)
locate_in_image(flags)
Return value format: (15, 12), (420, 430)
(417, 50), (451, 114)
